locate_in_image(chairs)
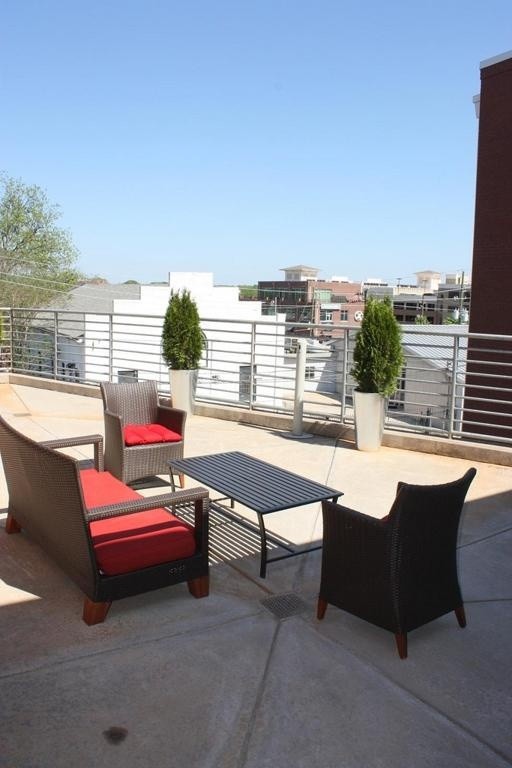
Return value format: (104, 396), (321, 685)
(97, 379), (187, 489)
(314, 463), (477, 662)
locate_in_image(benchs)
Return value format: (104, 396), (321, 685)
(2, 416), (212, 629)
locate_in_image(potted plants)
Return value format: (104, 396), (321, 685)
(162, 286), (207, 409)
(351, 293), (406, 452)
